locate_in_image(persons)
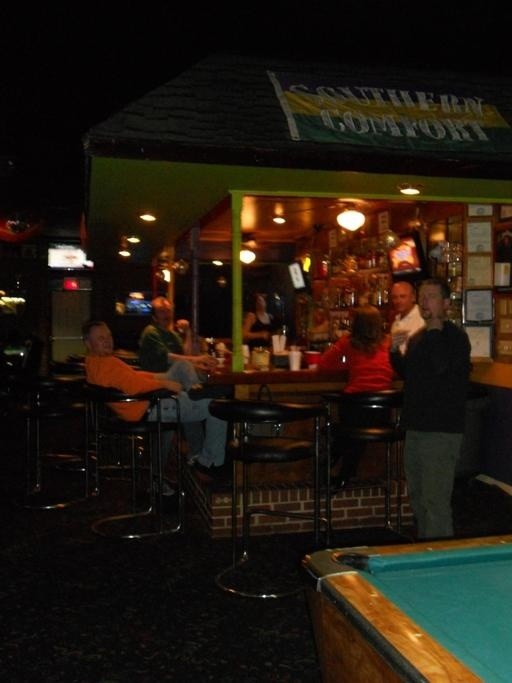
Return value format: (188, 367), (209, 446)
(387, 279), (425, 355)
(314, 301), (395, 481)
(242, 291), (278, 349)
(135, 296), (220, 499)
(385, 273), (473, 540)
(77, 317), (228, 475)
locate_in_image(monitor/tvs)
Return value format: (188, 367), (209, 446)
(386, 232), (427, 282)
(287, 261), (308, 291)
(42, 240), (93, 273)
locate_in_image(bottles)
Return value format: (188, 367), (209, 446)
(341, 250), (389, 330)
(208, 337), (216, 358)
(494, 235), (512, 286)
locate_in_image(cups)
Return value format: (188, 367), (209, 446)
(288, 352), (301, 371)
(271, 334), (286, 353)
(305, 351), (321, 370)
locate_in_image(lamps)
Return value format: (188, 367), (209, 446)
(336, 205), (365, 232)
(399, 183), (421, 196)
(240, 248), (256, 264)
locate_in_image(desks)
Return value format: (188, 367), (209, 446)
(299, 536), (512, 683)
